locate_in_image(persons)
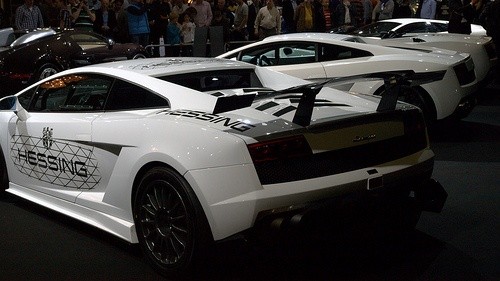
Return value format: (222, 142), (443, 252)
(282, 0), (299, 32)
(234, 0), (248, 40)
(15, 0), (44, 30)
(164, 0), (264, 57)
(67, 0), (96, 31)
(55, 0), (71, 29)
(96, 0), (117, 39)
(294, 0), (315, 32)
(315, 0), (500, 60)
(254, 0), (281, 39)
(114, 0), (128, 42)
(150, 0), (170, 44)
(127, 0), (150, 45)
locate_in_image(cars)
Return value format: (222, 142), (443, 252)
(0, 23), (150, 100)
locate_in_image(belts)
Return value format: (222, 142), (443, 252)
(259, 26), (275, 31)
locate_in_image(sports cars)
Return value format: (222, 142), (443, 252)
(217, 17), (498, 133)
(0, 57), (436, 275)
